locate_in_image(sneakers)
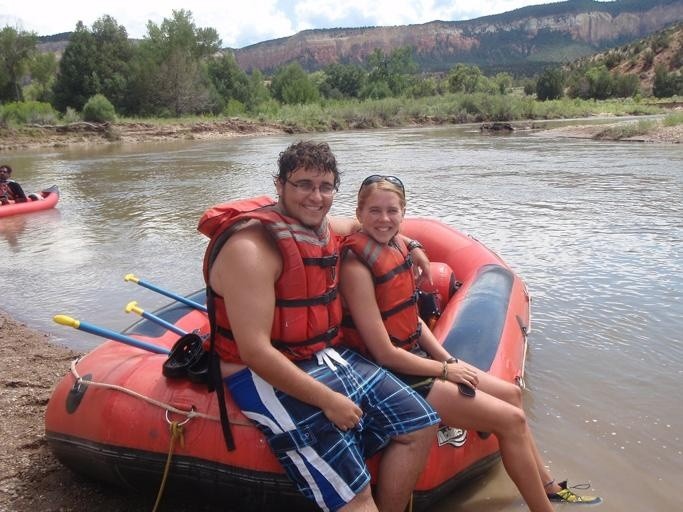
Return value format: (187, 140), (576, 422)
(546, 479), (602, 505)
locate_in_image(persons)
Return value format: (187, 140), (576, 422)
(339, 174), (603, 512)
(203, 141), (440, 511)
(0, 164), (28, 206)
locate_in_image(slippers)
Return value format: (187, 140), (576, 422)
(162, 333), (219, 383)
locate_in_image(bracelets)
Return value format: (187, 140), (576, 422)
(447, 357), (458, 363)
(407, 240), (422, 251)
(441, 361), (447, 378)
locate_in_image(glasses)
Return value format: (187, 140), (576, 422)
(358, 175), (406, 199)
(285, 178), (339, 199)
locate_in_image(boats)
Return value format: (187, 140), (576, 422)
(45, 218), (531, 511)
(0, 185), (59, 217)
